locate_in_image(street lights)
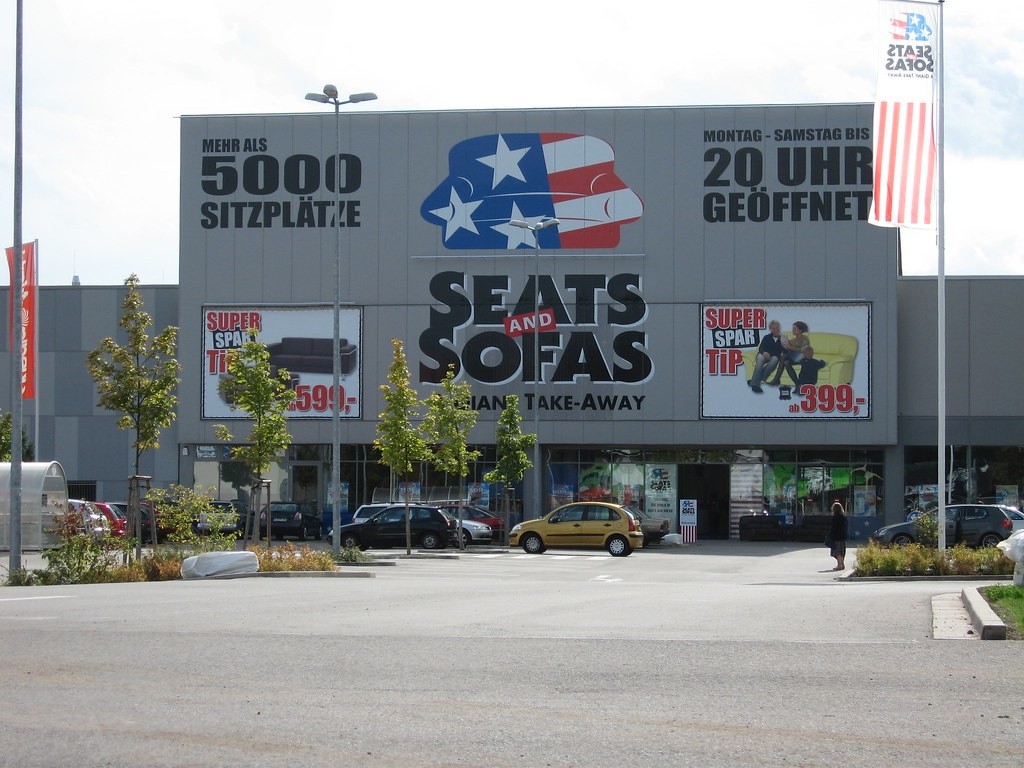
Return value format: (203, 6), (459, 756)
(305, 82), (378, 557)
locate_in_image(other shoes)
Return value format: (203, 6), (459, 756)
(833, 566), (845, 570)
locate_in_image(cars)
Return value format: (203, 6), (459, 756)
(872, 504), (1013, 550)
(434, 505), (504, 537)
(799, 489), (849, 513)
(1001, 505), (1024, 532)
(65, 500), (178, 549)
(507, 502), (645, 558)
(556, 506), (672, 545)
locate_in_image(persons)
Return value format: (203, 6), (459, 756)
(824, 502), (848, 570)
(747, 320), (825, 396)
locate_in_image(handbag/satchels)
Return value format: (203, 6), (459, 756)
(824, 529), (835, 548)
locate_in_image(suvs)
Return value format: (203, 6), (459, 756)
(196, 499), (255, 540)
(352, 500), (493, 549)
(326, 501), (458, 551)
(257, 500), (326, 543)
